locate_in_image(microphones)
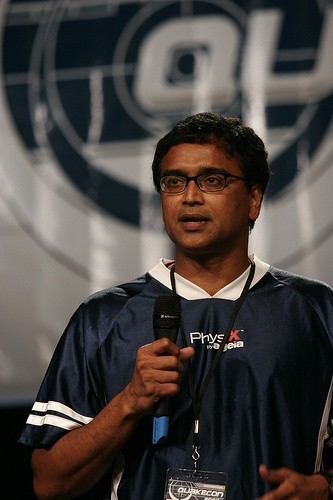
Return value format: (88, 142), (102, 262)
(152, 295), (181, 448)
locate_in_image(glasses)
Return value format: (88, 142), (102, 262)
(157, 172), (246, 193)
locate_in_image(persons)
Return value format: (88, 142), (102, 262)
(19, 112), (333, 499)
